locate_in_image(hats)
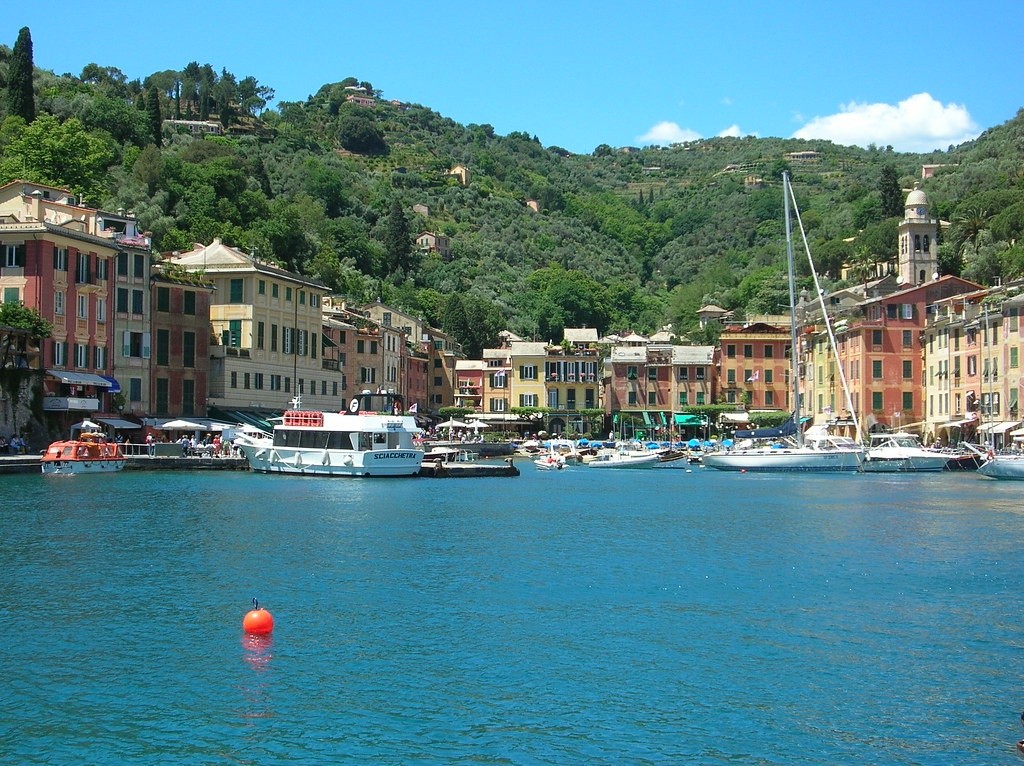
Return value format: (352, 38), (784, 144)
(207, 434), (211, 436)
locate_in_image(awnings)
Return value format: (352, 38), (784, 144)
(939, 419), (1024, 434)
(99, 418), (141, 429)
(674, 414), (702, 426)
(803, 426), (824, 435)
(799, 417), (811, 424)
(48, 371), (120, 392)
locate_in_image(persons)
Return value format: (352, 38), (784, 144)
(548, 456), (563, 468)
(532, 433), (563, 440)
(0, 433), (233, 459)
(375, 434), (386, 444)
(675, 435), (680, 441)
(433, 463), (441, 475)
(394, 406), (399, 415)
(609, 430), (614, 441)
(414, 427), (480, 442)
(932, 436), (1024, 454)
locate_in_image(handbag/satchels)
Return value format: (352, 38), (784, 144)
(146, 436), (150, 443)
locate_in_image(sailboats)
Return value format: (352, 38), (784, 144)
(511, 171), (1024, 482)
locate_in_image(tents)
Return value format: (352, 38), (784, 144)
(772, 443), (787, 449)
(545, 442), (558, 448)
(579, 438), (733, 450)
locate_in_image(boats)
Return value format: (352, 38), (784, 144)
(39, 431), (128, 476)
(423, 446), (460, 462)
(232, 385), (425, 477)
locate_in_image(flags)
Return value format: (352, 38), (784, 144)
(495, 369), (504, 376)
(410, 404), (417, 413)
(747, 371), (759, 381)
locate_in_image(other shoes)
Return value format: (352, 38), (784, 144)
(216, 456), (220, 458)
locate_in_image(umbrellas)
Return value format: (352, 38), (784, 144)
(433, 457), (443, 461)
(154, 420), (207, 430)
(435, 420), (490, 429)
(234, 424), (264, 432)
